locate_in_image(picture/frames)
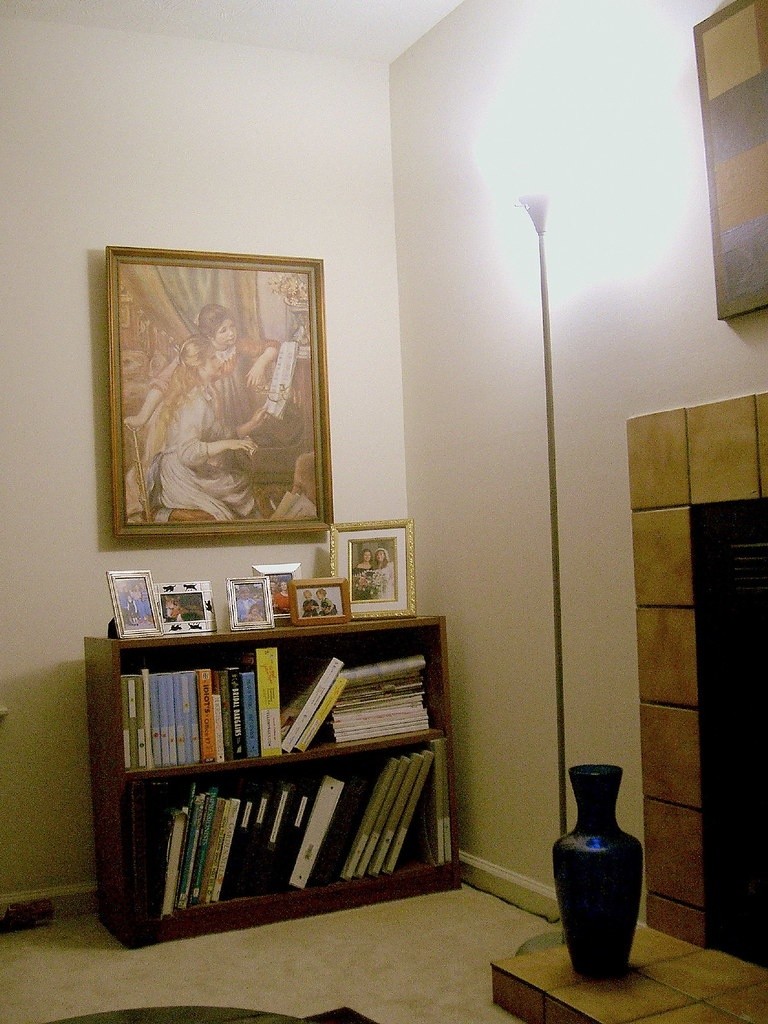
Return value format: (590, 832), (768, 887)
(252, 563), (303, 620)
(106, 570), (165, 639)
(286, 577), (352, 626)
(330, 518), (417, 621)
(106, 246), (335, 536)
(153, 580), (217, 634)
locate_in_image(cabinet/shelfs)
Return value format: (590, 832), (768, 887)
(85, 614), (462, 951)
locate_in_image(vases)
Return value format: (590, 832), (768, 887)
(226, 576), (275, 631)
(553, 765), (645, 981)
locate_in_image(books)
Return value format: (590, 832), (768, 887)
(121, 644), (453, 918)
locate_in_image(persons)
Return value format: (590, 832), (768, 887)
(123, 547), (395, 627)
